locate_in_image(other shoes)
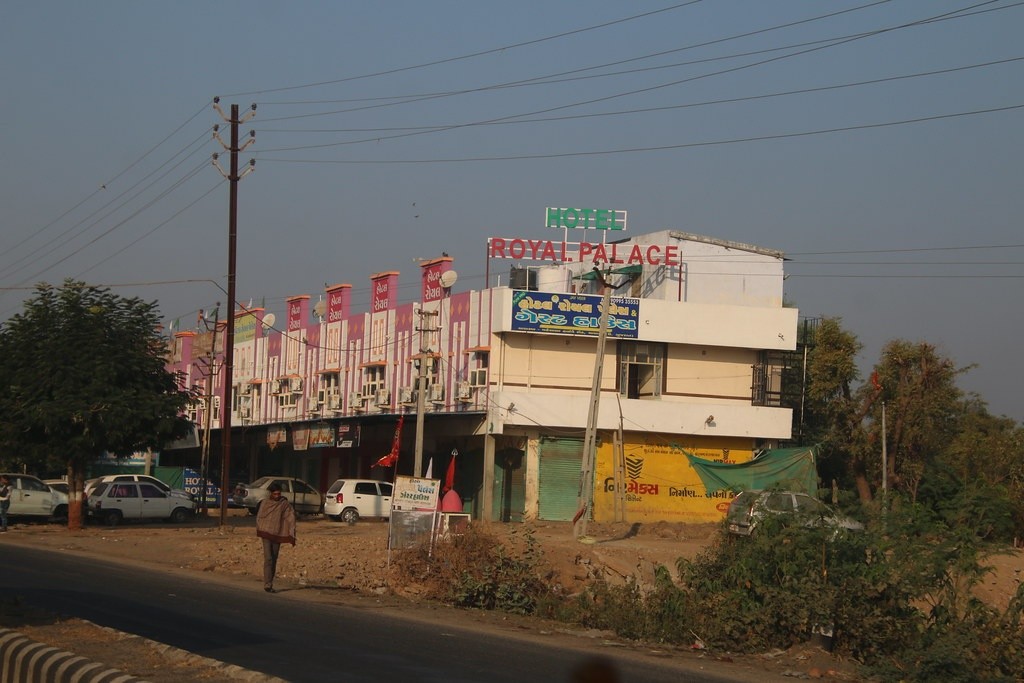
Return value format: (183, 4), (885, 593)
(264, 584), (272, 592)
(0, 528), (7, 532)
(271, 590), (276, 593)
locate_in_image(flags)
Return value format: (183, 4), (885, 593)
(370, 418), (403, 469)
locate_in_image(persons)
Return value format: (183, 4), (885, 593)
(0, 474), (13, 532)
(256, 484), (296, 592)
(352, 426), (360, 447)
(81, 488), (89, 526)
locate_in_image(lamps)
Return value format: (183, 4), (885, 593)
(706, 415), (714, 425)
(508, 403), (515, 411)
(414, 358), (420, 367)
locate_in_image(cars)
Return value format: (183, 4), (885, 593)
(87, 474), (195, 500)
(43, 479), (85, 501)
(0, 472), (69, 523)
(232, 475), (322, 515)
(323, 479), (393, 523)
(84, 478), (102, 496)
(89, 481), (199, 526)
(726, 489), (868, 543)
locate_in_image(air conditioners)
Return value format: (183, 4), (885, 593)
(238, 382), (250, 395)
(427, 383), (442, 401)
(374, 389), (389, 405)
(200, 400), (207, 409)
(188, 402), (196, 410)
(347, 392), (362, 408)
(288, 377), (301, 391)
(213, 396), (220, 407)
(306, 397), (318, 411)
(327, 395), (340, 409)
(238, 404), (248, 417)
(396, 386), (412, 403)
(268, 380), (280, 394)
(454, 382), (469, 398)
